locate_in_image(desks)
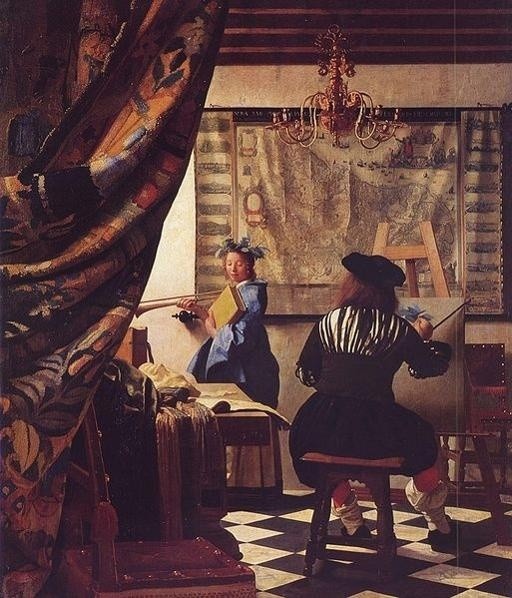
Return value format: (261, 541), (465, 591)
(196, 382), (271, 445)
(297, 451), (406, 584)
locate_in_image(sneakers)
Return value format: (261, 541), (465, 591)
(340, 524), (371, 538)
(428, 516), (460, 550)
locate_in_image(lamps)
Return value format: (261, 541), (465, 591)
(265, 22), (408, 150)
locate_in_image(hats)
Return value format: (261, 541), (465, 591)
(341, 253), (406, 288)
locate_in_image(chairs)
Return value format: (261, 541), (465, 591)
(58, 400), (256, 597)
